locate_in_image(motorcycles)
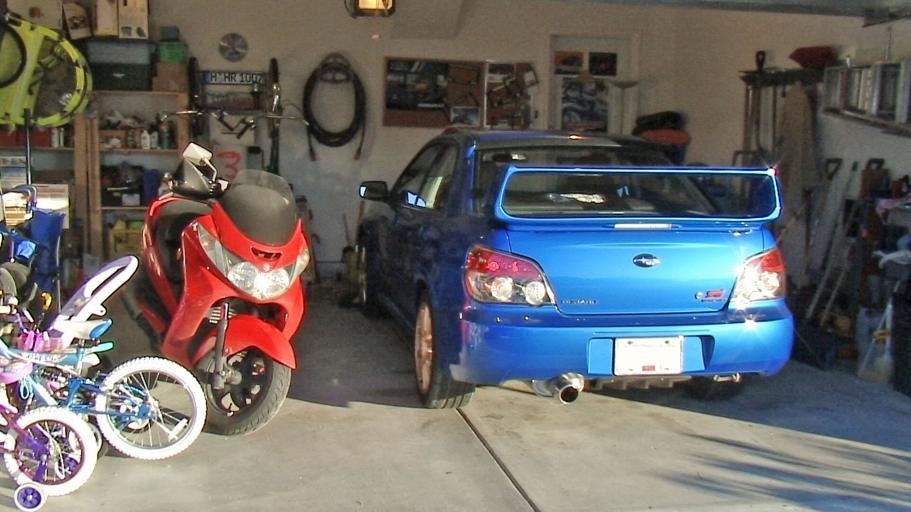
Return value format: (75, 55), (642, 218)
(120, 141), (311, 438)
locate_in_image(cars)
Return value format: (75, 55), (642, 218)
(351, 125), (800, 408)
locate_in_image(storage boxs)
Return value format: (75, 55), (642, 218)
(119, 0), (149, 39)
(94, 1), (119, 37)
(152, 63), (190, 91)
(84, 37), (153, 91)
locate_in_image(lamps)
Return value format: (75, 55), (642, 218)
(344, 0), (396, 19)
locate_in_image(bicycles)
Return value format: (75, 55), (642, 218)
(0, 251), (208, 512)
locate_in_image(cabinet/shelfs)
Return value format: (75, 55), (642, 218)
(88, 90), (190, 272)
(1, 112), (90, 254)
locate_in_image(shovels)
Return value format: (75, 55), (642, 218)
(790, 157), (885, 371)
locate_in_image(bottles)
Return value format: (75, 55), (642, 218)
(126, 124), (171, 151)
(51, 126), (65, 148)
